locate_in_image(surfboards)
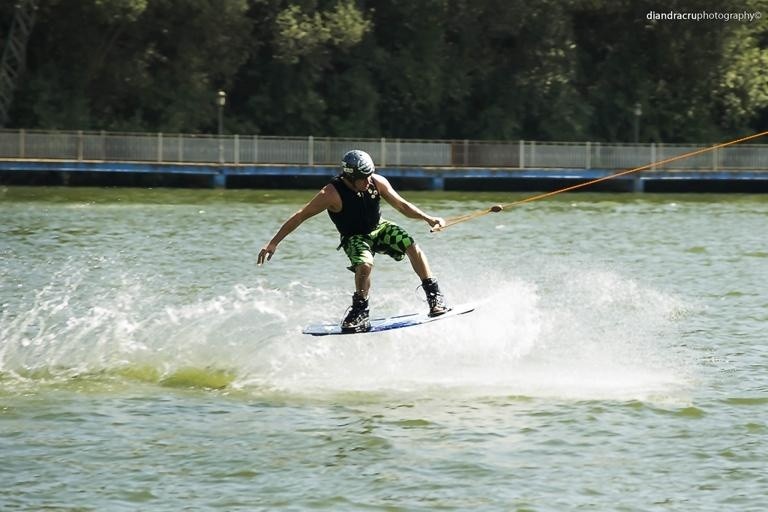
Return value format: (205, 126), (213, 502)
(301, 304), (474, 336)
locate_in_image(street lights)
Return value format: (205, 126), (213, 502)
(214, 90), (227, 165)
(635, 102), (642, 147)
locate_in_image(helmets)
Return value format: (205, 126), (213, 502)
(341, 149), (375, 183)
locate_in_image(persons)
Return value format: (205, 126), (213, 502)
(256, 150), (446, 330)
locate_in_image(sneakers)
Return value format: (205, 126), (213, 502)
(340, 295), (370, 330)
(421, 277), (447, 316)
